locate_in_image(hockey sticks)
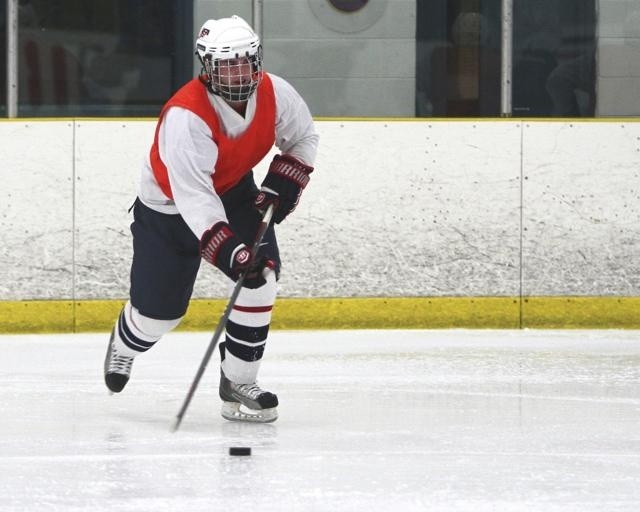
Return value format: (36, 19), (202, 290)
(139, 203), (276, 445)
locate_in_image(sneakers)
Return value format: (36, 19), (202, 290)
(104, 326), (134, 392)
(219, 366), (278, 410)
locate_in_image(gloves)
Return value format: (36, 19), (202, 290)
(199, 221), (272, 288)
(253, 154), (314, 223)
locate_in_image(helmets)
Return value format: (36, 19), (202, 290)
(195, 15), (264, 103)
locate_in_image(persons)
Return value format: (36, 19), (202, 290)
(103, 14), (319, 411)
